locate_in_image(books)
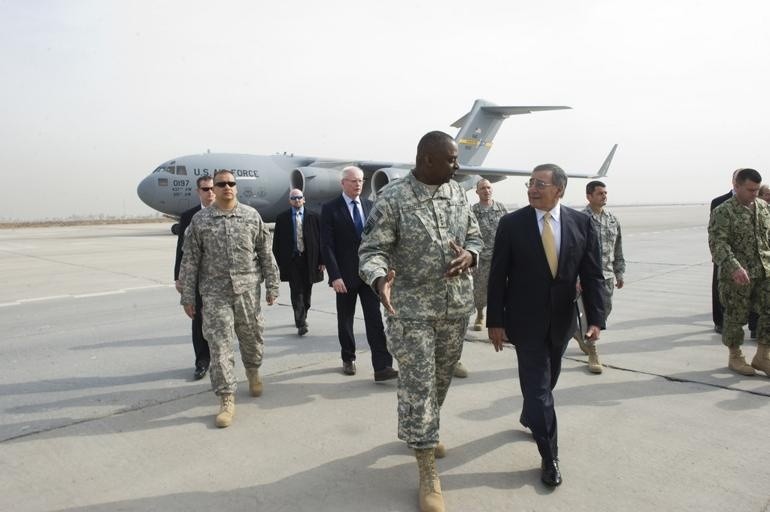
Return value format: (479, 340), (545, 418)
(573, 292), (590, 344)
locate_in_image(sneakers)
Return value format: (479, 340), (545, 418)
(454, 359), (468, 378)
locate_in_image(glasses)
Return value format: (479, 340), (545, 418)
(291, 196), (302, 200)
(347, 180), (365, 183)
(215, 181), (236, 187)
(525, 182), (553, 189)
(201, 187), (212, 191)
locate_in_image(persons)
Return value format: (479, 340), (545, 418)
(486, 164), (606, 486)
(710, 168), (758, 338)
(358, 131), (486, 511)
(759, 185), (770, 201)
(578, 182), (626, 372)
(273, 189), (325, 338)
(320, 165), (399, 381)
(175, 176), (215, 380)
(454, 362), (468, 377)
(708, 169), (770, 378)
(467, 178), (509, 331)
(178, 168), (280, 429)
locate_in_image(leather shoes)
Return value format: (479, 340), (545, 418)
(299, 326), (307, 334)
(715, 325), (722, 333)
(194, 367), (207, 378)
(375, 367), (398, 381)
(344, 360), (355, 374)
(541, 457), (562, 486)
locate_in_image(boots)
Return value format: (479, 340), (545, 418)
(574, 328), (602, 373)
(216, 393), (234, 427)
(416, 444), (445, 512)
(728, 344), (754, 375)
(246, 367), (262, 396)
(751, 343), (770, 375)
(474, 309), (484, 332)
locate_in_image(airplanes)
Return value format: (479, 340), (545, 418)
(136, 100), (618, 223)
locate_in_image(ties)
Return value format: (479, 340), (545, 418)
(542, 213), (558, 279)
(352, 200), (363, 239)
(295, 210), (304, 252)
